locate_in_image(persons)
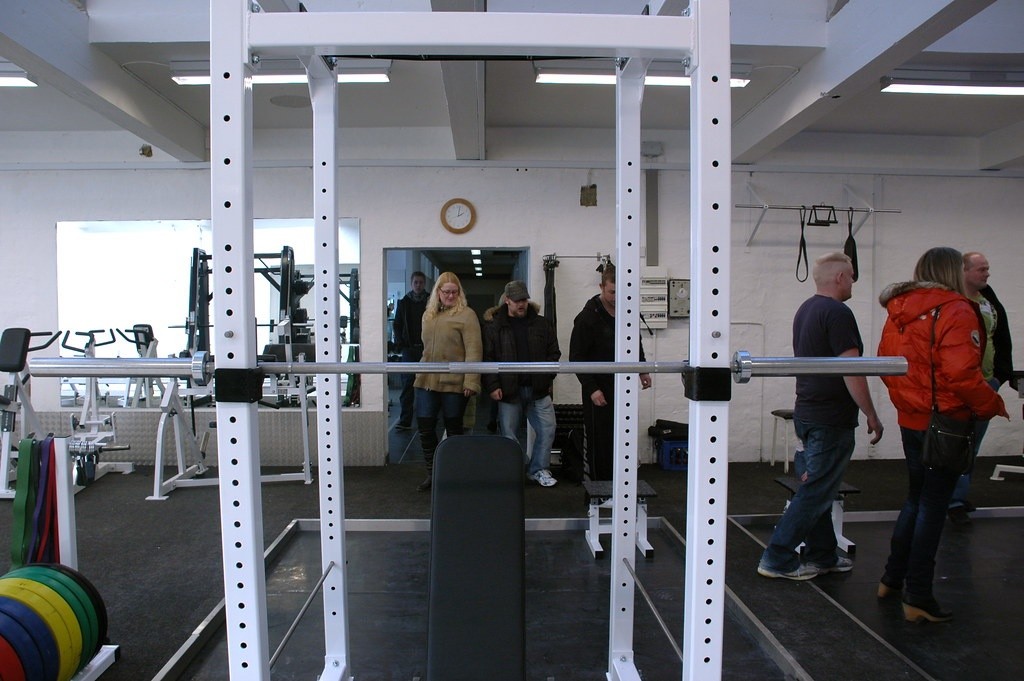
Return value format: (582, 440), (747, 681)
(392, 271), (431, 432)
(486, 292), (509, 434)
(946, 253), (1014, 529)
(480, 281), (563, 487)
(387, 303), (394, 317)
(757, 251), (885, 582)
(414, 272), (483, 490)
(569, 264), (653, 508)
(876, 246), (1011, 627)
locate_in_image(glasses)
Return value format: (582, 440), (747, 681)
(438, 287), (460, 295)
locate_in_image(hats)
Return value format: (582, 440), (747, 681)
(506, 281), (529, 302)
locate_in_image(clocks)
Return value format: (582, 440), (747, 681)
(440, 198), (476, 235)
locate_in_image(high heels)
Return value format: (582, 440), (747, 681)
(878, 581), (904, 599)
(904, 593), (952, 624)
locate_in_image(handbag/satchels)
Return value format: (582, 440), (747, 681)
(920, 412), (975, 473)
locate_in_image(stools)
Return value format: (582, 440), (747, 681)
(580, 480), (654, 559)
(770, 409), (794, 474)
(773, 478), (861, 557)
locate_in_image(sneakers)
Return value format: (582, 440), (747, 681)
(807, 556), (855, 575)
(527, 467), (558, 488)
(759, 562), (819, 581)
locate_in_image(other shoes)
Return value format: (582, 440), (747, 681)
(947, 504), (971, 527)
(396, 421), (411, 430)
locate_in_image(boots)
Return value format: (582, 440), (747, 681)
(417, 417), (437, 492)
(442, 416), (464, 437)
